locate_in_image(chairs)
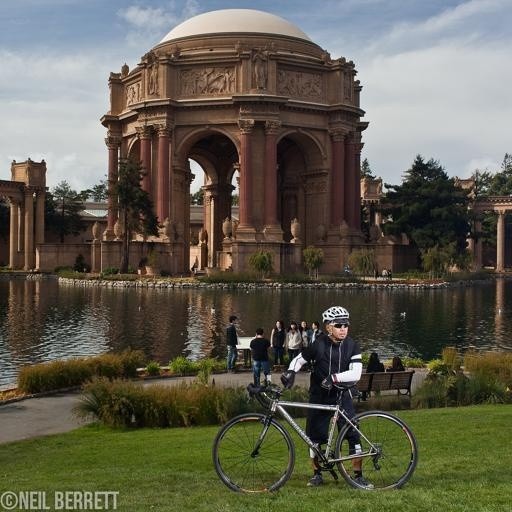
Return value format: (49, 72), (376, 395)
(354, 476), (374, 489)
(307, 474), (323, 487)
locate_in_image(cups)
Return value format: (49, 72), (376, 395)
(330, 323), (349, 328)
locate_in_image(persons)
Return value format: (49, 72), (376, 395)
(146, 58), (158, 94)
(386, 356), (405, 371)
(388, 269), (392, 280)
(281, 305), (374, 490)
(225, 315), (324, 388)
(252, 47), (266, 90)
(382, 267), (388, 280)
(362, 352), (385, 401)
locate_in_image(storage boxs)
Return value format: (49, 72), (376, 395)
(359, 370), (415, 396)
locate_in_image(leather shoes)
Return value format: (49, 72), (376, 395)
(321, 375), (333, 391)
(281, 369), (295, 389)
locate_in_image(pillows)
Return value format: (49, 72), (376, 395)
(322, 306), (350, 324)
